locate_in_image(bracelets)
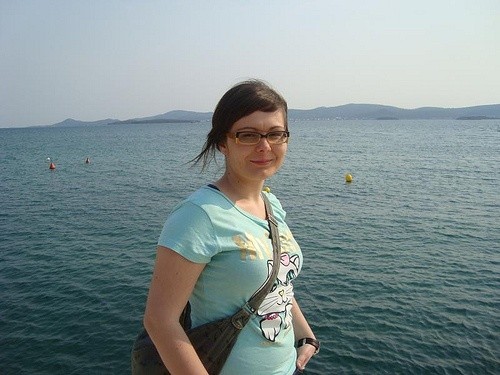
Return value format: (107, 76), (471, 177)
(296, 338), (320, 356)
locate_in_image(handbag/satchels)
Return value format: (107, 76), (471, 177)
(131, 300), (253, 375)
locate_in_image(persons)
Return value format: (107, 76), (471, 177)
(143, 79), (320, 375)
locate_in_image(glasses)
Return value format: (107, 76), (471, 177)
(224, 130), (289, 146)
